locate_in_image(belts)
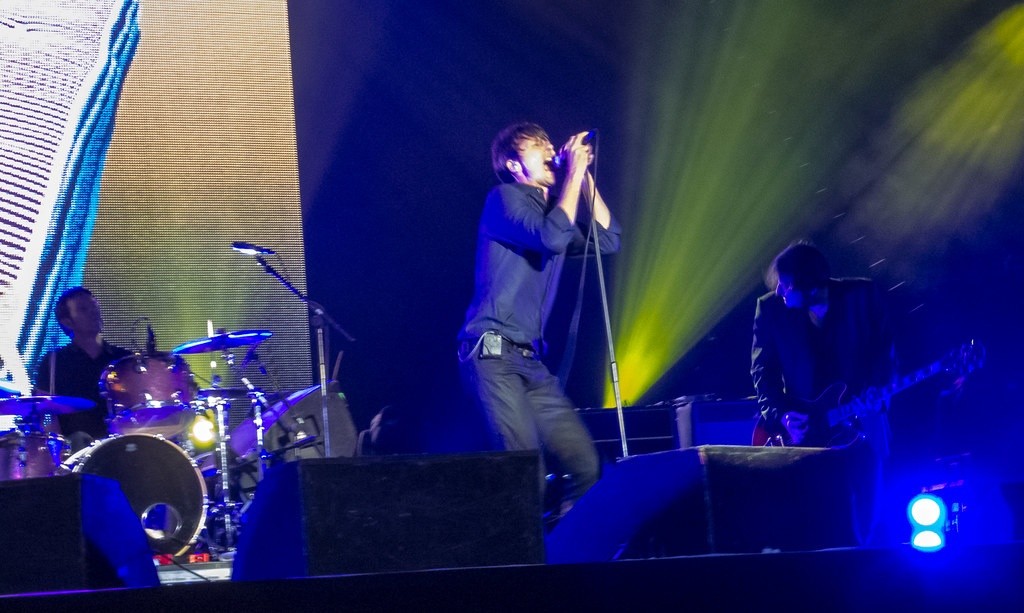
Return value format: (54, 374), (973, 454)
(513, 344), (535, 358)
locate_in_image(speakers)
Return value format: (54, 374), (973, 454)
(570, 405), (680, 479)
(227, 381), (358, 503)
(0, 472), (161, 598)
(231, 447), (545, 581)
(545, 443), (860, 564)
(673, 399), (764, 449)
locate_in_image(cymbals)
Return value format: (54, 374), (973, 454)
(0, 396), (99, 416)
(192, 387), (259, 404)
(172, 328), (273, 356)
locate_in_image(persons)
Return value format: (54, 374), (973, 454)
(458, 124), (622, 518)
(751, 243), (964, 551)
(30, 286), (134, 457)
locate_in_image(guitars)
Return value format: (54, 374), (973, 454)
(751, 336), (988, 451)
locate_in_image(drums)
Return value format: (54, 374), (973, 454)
(57, 432), (211, 563)
(96, 350), (198, 442)
(0, 430), (67, 481)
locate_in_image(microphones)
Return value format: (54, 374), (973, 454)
(146, 325), (156, 352)
(549, 130), (599, 171)
(232, 241), (276, 255)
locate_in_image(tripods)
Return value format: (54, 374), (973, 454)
(196, 394), (243, 564)
